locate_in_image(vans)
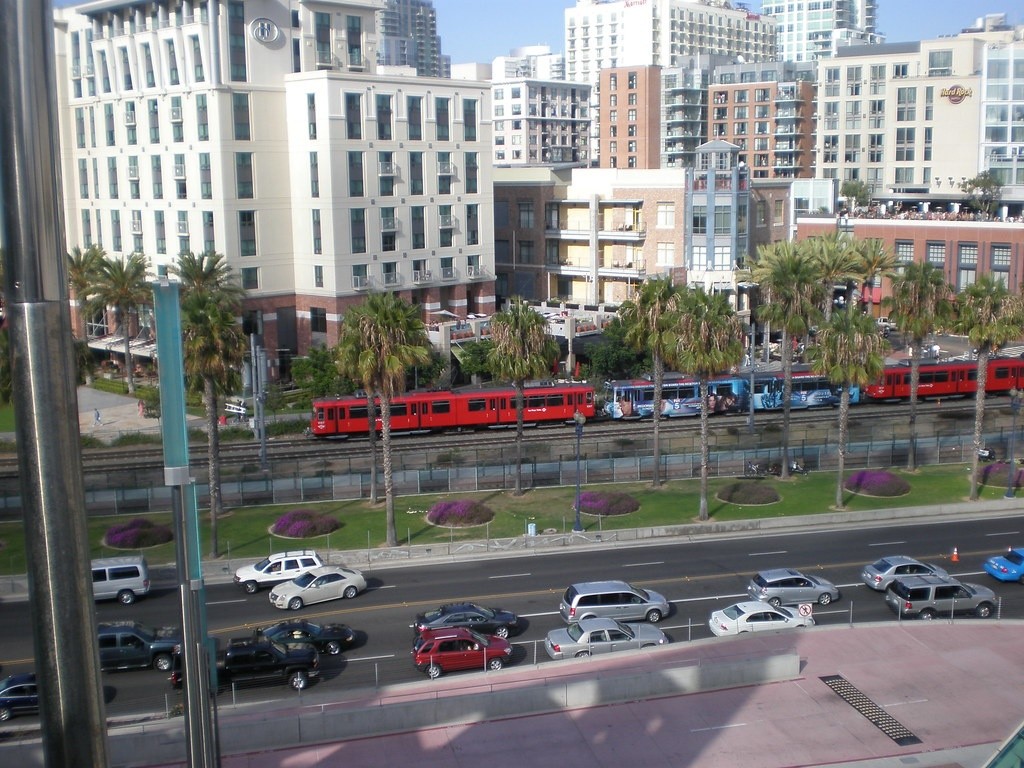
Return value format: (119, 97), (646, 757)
(91, 555), (151, 605)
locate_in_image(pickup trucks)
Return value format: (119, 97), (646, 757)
(171, 635), (321, 697)
(876, 318), (897, 329)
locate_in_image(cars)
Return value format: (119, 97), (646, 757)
(544, 618), (670, 660)
(268, 561), (367, 610)
(708, 568), (840, 637)
(984, 548), (1024, 584)
(0, 670), (39, 722)
(861, 555), (950, 589)
(254, 619), (356, 655)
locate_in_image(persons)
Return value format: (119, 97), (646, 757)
(745, 348), (751, 367)
(706, 394), (721, 414)
(906, 342), (955, 362)
(219, 413), (227, 430)
(235, 399), (247, 422)
(92, 409), (103, 427)
(136, 364), (142, 376)
(650, 400), (667, 415)
(619, 396), (641, 418)
(138, 400), (146, 418)
(717, 392), (738, 412)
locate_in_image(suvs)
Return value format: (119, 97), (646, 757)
(232, 550), (323, 595)
(559, 580), (670, 626)
(412, 599), (517, 679)
(885, 575), (997, 620)
(96, 620), (180, 672)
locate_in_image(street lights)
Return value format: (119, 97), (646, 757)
(1007, 386), (1023, 499)
(573, 410), (584, 533)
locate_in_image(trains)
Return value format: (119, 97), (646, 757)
(309, 360), (1024, 440)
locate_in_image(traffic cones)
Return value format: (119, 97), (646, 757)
(1008, 545), (1011, 551)
(951, 546), (959, 561)
